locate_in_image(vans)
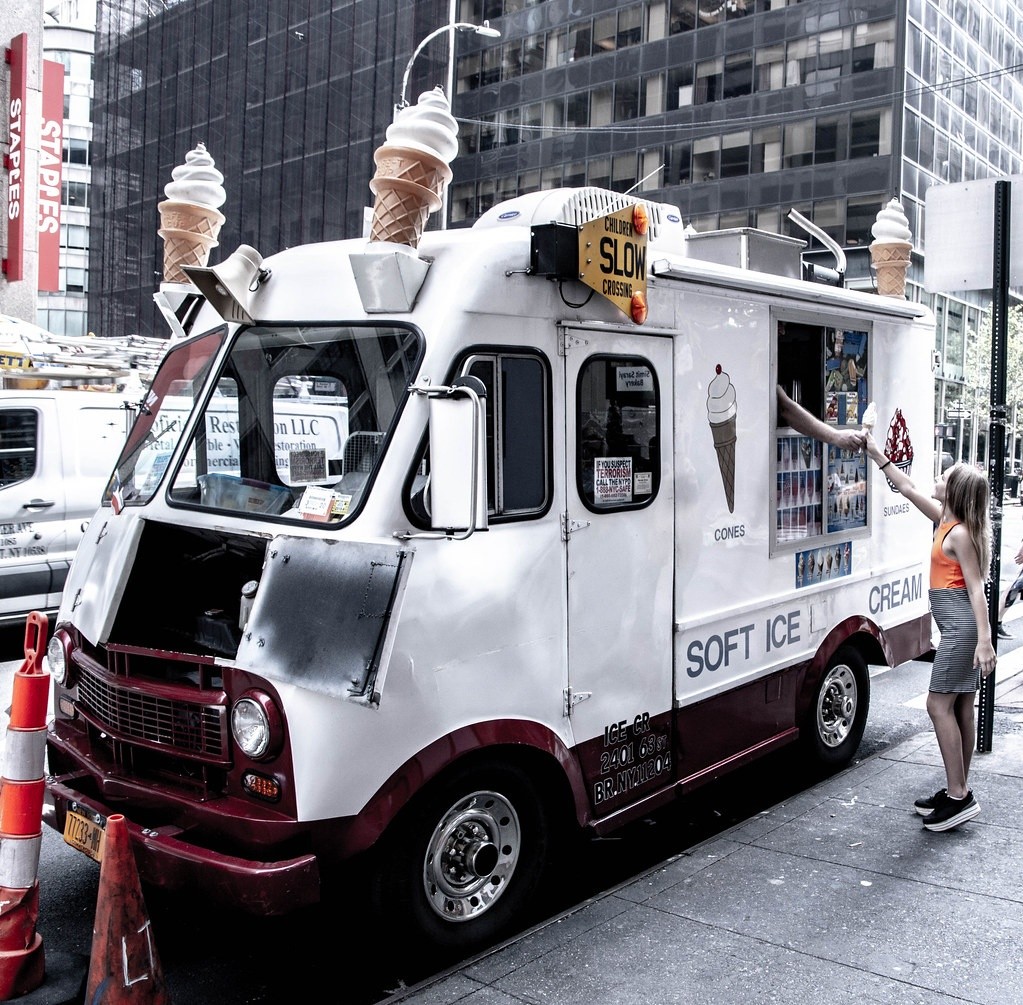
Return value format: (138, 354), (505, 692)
(0, 388), (350, 663)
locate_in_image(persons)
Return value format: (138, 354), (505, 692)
(998, 539), (1023, 640)
(862, 429), (998, 831)
(776, 385), (867, 453)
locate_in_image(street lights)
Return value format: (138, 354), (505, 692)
(401, 24), (503, 105)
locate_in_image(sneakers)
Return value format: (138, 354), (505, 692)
(913, 788), (948, 817)
(922, 788), (982, 832)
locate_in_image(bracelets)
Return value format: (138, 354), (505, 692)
(879, 461), (890, 469)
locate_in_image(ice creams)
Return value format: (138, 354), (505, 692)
(369, 86), (458, 248)
(157, 143), (226, 285)
(868, 196), (914, 302)
(861, 401), (878, 434)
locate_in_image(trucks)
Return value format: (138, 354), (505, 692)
(42, 86), (942, 977)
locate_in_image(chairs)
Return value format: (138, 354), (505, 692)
(342, 432), (427, 479)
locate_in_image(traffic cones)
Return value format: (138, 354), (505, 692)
(83, 813), (168, 1005)
